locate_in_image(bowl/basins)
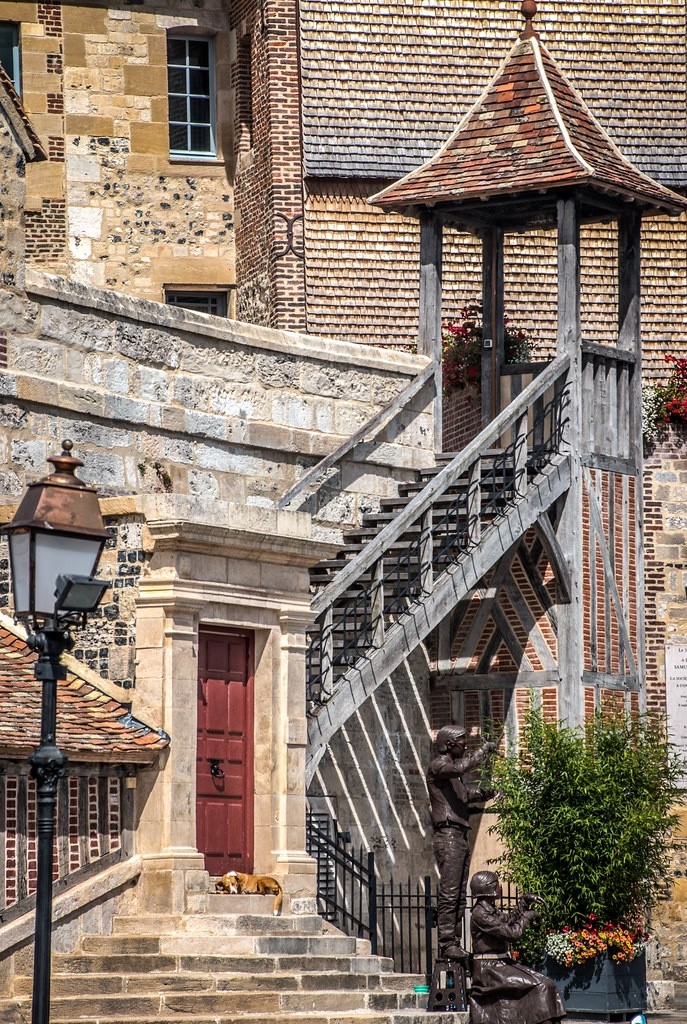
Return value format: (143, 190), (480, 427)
(412, 985), (430, 993)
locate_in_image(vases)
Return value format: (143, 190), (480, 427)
(532, 949), (648, 1013)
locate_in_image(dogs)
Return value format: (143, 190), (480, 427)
(211, 871), (283, 916)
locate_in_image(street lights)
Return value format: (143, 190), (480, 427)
(1, 440), (113, 1024)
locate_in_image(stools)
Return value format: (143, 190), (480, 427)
(427, 958), (468, 1012)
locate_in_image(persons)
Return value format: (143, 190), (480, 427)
(426, 724), (506, 959)
(465, 871), (567, 1024)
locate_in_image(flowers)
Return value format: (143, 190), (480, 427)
(471, 684), (687, 970)
(641, 356), (687, 444)
(404, 297), (538, 411)
